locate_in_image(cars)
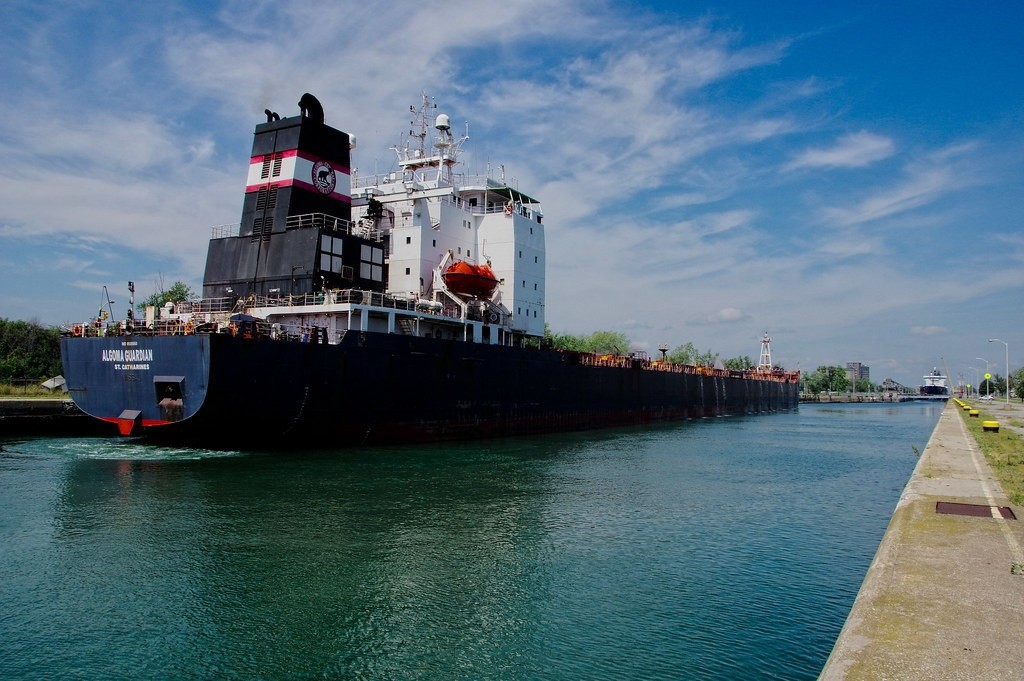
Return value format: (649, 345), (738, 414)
(978, 393), (995, 400)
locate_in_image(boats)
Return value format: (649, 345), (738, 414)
(914, 366), (949, 401)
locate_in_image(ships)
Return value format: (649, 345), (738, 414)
(56, 93), (802, 456)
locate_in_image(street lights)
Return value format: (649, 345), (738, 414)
(969, 366), (979, 400)
(850, 364), (855, 394)
(976, 356), (990, 403)
(988, 337), (1010, 404)
